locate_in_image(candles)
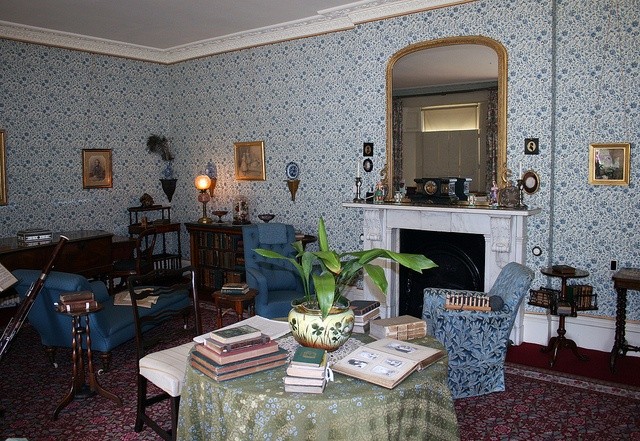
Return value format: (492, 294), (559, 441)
(356, 159), (360, 178)
(519, 159), (522, 179)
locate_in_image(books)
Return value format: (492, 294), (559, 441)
(57, 299), (99, 313)
(196, 266), (246, 290)
(348, 300), (382, 335)
(191, 314), (291, 349)
(196, 249), (245, 269)
(329, 336), (448, 391)
(284, 347), (333, 396)
(221, 282), (250, 296)
(197, 231), (244, 249)
(368, 315), (427, 344)
(0, 263), (19, 294)
(566, 285), (594, 309)
(189, 323), (290, 384)
(552, 265), (577, 275)
(153, 258), (180, 274)
(58, 292), (95, 305)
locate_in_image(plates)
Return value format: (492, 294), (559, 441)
(285, 160), (300, 179)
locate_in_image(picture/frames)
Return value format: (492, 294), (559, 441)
(588, 143), (631, 186)
(83, 148), (113, 188)
(234, 140), (265, 179)
(0, 127), (8, 206)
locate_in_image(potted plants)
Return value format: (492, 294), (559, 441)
(252, 211), (440, 355)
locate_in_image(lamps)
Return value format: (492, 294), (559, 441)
(195, 172), (212, 223)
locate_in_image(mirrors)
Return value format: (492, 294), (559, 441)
(384, 35), (507, 207)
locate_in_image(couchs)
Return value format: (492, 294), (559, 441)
(242, 218), (314, 322)
(419, 260), (532, 397)
(11, 264), (195, 373)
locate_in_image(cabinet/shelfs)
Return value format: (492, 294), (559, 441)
(184, 221), (244, 294)
(128, 203), (184, 279)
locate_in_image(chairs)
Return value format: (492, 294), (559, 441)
(125, 264), (205, 440)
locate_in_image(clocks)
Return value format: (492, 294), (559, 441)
(425, 180), (437, 195)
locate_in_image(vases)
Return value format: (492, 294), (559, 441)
(163, 161), (175, 179)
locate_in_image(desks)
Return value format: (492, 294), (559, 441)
(45, 290), (126, 420)
(174, 328), (460, 441)
(525, 260), (597, 370)
(607, 265), (640, 373)
(212, 287), (260, 330)
(112, 234), (140, 292)
(0, 228), (115, 302)
